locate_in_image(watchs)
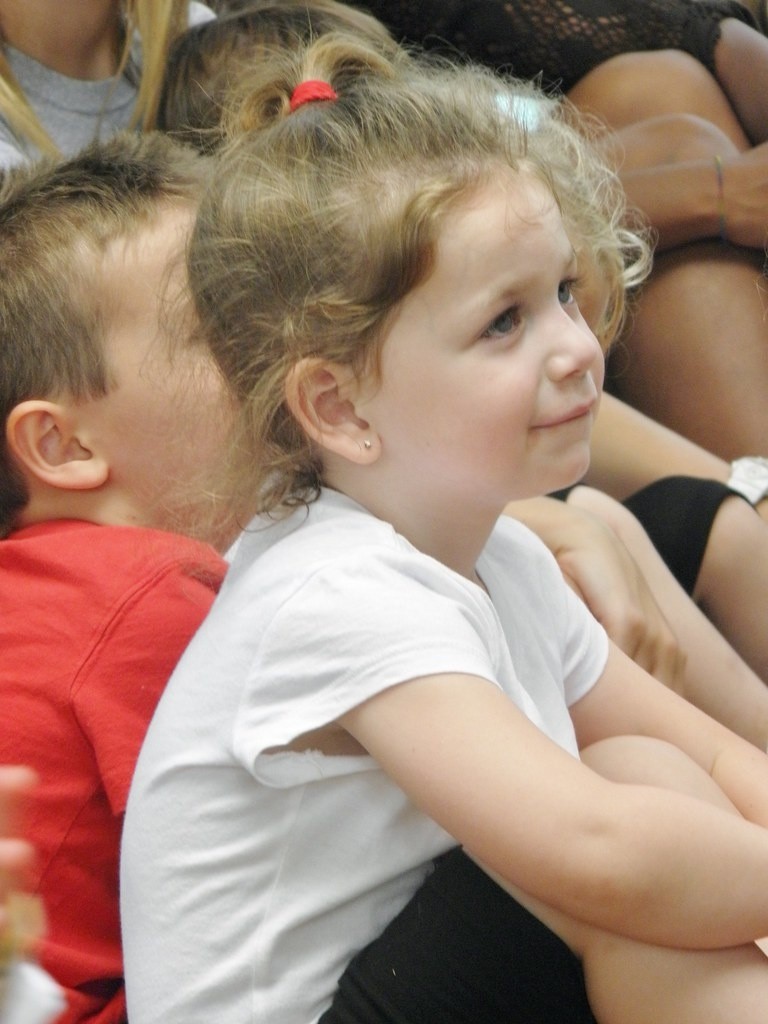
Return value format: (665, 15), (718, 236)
(720, 447), (767, 506)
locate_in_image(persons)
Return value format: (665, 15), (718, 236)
(151, 2), (763, 688)
(122, 35), (767, 1024)
(0, 132), (683, 1024)
(0, 0), (226, 185)
(362, 0), (763, 465)
